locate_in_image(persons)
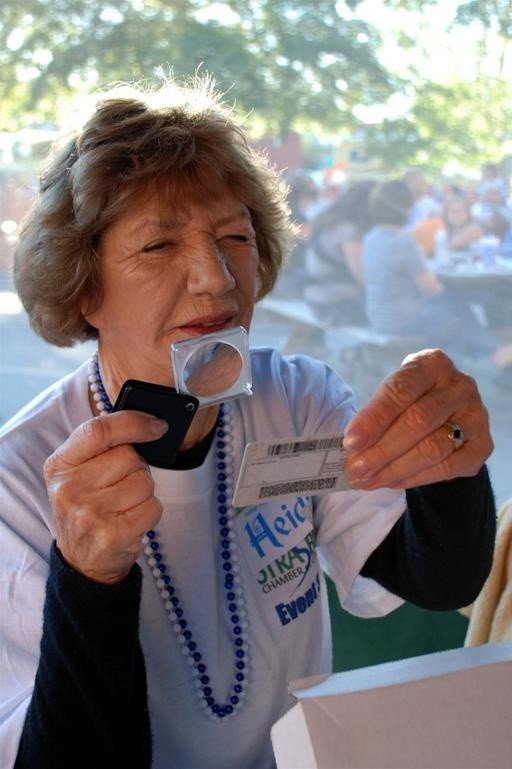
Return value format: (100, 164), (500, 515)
(0, 65), (496, 769)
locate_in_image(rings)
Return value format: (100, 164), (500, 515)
(447, 422), (464, 449)
